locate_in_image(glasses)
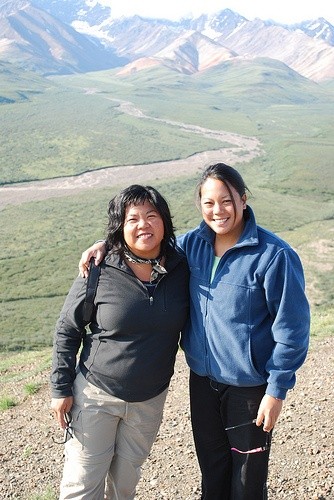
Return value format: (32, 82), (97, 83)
(226, 419), (270, 454)
(51, 410), (74, 445)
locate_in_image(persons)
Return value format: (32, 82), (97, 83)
(79, 163), (311, 500)
(52, 184), (189, 500)
